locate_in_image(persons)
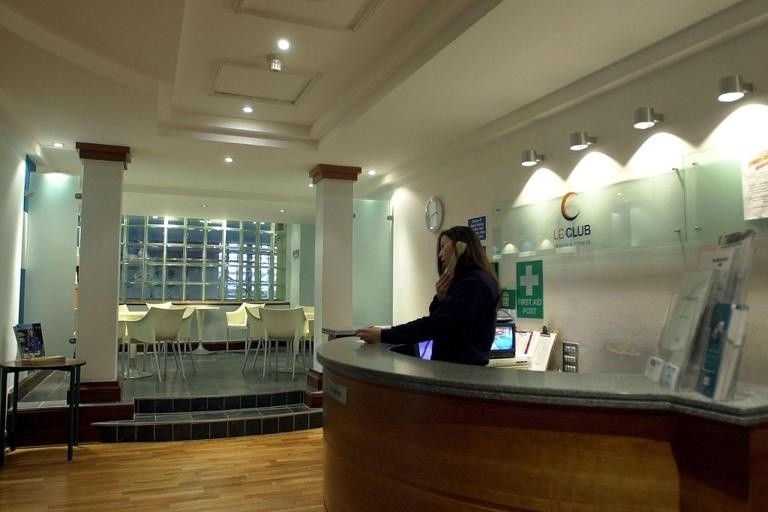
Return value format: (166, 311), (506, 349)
(357, 225), (500, 366)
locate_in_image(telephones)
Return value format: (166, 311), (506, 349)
(443, 241), (467, 275)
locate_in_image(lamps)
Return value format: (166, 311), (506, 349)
(633, 102), (664, 131)
(569, 128), (596, 152)
(716, 74), (757, 106)
(263, 55), (285, 72)
(519, 149), (545, 171)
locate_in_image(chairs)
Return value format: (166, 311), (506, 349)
(117, 295), (315, 383)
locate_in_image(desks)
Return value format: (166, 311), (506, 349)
(0, 356), (86, 461)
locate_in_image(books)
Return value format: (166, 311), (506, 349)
(13, 323), (66, 365)
(698, 304), (750, 399)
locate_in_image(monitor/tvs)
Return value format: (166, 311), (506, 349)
(417, 338), (435, 364)
(490, 323), (518, 357)
